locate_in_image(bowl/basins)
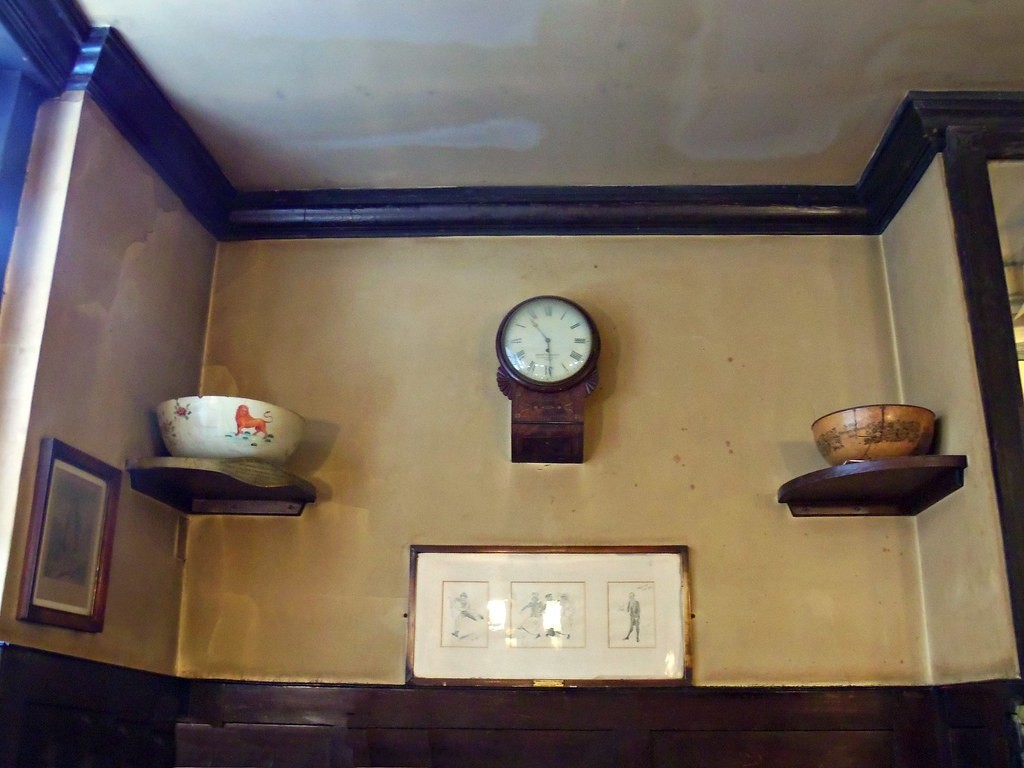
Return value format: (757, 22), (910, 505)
(152, 396), (307, 466)
(810, 404), (935, 465)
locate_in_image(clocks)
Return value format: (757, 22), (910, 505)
(494, 294), (601, 463)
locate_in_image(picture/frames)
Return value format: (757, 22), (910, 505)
(14, 437), (124, 632)
(404, 544), (694, 686)
